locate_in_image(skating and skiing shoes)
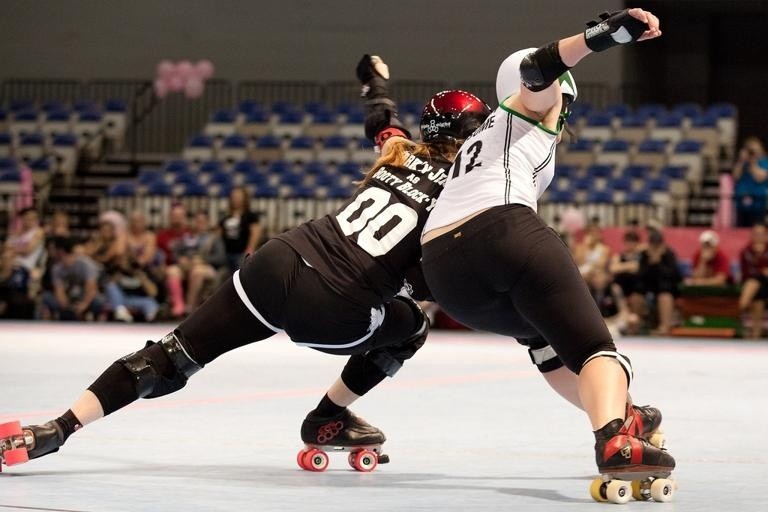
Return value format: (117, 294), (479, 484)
(591, 414), (675, 504)
(297, 409), (389, 472)
(625, 392), (666, 450)
(0, 420), (64, 473)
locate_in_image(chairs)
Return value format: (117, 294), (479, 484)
(110, 98), (738, 216)
(1, 95), (129, 199)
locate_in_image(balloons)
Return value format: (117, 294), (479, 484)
(154, 57), (215, 101)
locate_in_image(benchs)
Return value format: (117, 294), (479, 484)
(583, 263), (767, 337)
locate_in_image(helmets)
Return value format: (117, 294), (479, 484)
(420, 90), (492, 148)
(496, 48), (578, 106)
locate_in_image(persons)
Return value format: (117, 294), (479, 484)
(204, 187), (262, 273)
(732, 135), (765, 224)
(0, 202), (225, 323)
(420, 8), (676, 474)
(548, 212), (768, 337)
(23, 53), (487, 445)
